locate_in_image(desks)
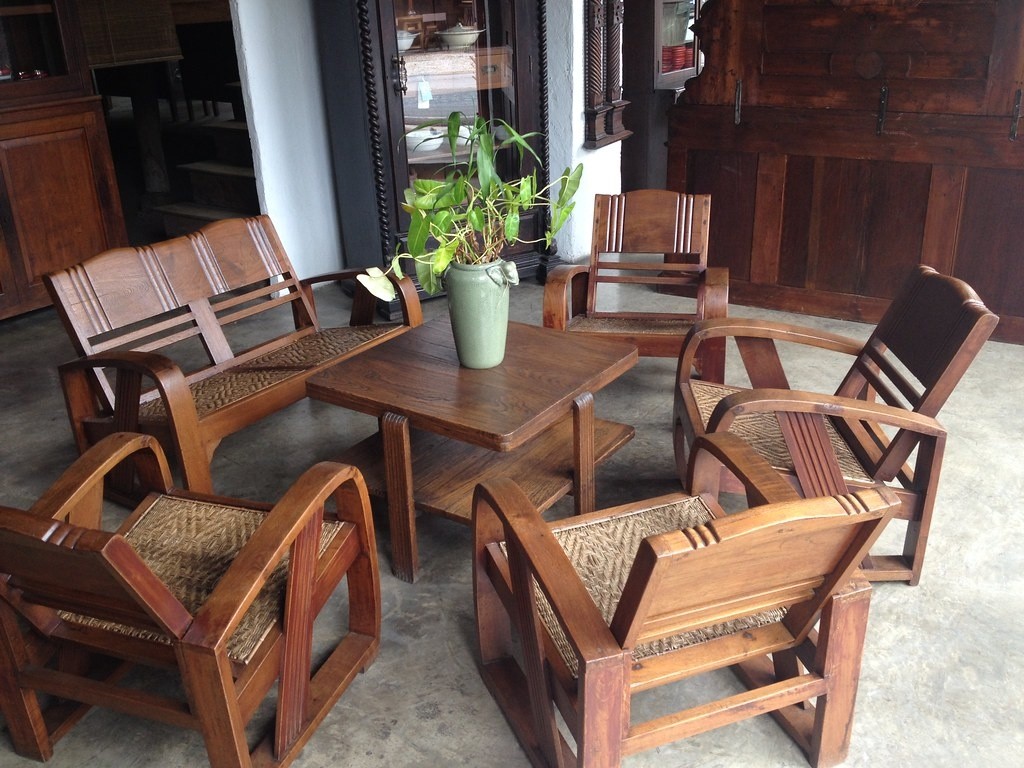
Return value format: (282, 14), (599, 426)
(304, 313), (639, 585)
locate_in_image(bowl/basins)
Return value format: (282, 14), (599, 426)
(431, 125), (478, 146)
(397, 30), (420, 53)
(434, 23), (485, 49)
(406, 131), (444, 153)
(661, 45), (693, 74)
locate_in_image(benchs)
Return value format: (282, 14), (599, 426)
(42, 214), (423, 498)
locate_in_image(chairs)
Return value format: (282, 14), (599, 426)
(669, 262), (1000, 585)
(542, 189), (731, 383)
(470, 429), (902, 768)
(0, 431), (383, 768)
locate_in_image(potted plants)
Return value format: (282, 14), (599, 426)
(357, 106), (586, 366)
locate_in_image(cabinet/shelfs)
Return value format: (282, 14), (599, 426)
(309, 0), (573, 323)
(0, 0), (133, 319)
